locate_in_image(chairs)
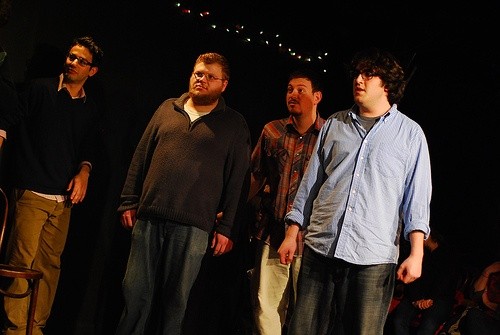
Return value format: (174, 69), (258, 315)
(0, 187), (43, 335)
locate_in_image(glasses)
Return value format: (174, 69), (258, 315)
(192, 70), (224, 83)
(67, 52), (92, 68)
(352, 68), (381, 82)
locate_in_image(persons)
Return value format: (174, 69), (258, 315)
(390, 228), (500, 335)
(115, 53), (250, 335)
(277, 50), (433, 335)
(0, 36), (109, 335)
(215, 70), (330, 335)
